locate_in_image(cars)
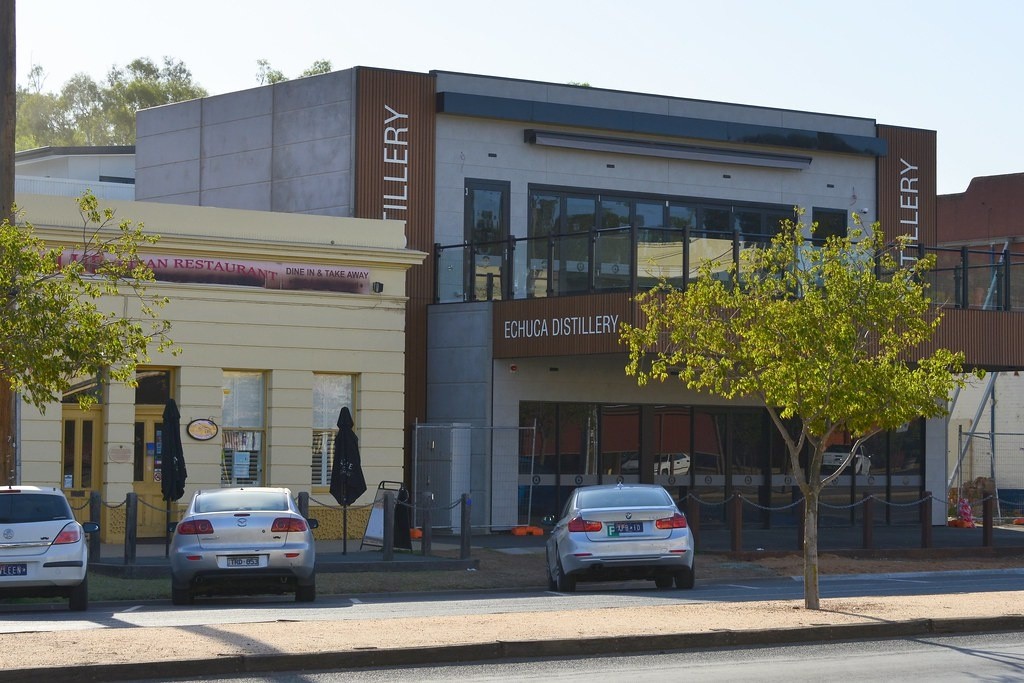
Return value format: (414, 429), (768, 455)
(167, 486), (318, 606)
(618, 451), (691, 477)
(541, 481), (694, 593)
(819, 443), (875, 476)
(0, 485), (100, 612)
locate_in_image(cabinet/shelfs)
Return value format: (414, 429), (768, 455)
(413, 423), (472, 537)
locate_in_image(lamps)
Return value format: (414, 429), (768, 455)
(371, 282), (384, 294)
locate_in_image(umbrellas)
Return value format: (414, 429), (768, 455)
(328, 405), (368, 559)
(153, 395), (188, 564)
